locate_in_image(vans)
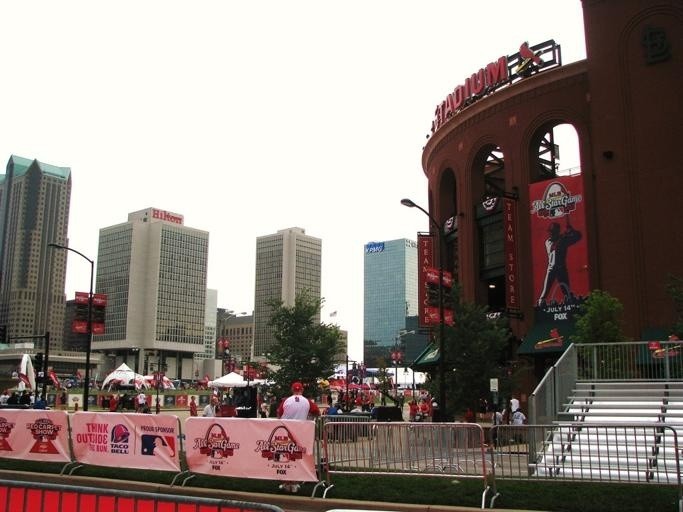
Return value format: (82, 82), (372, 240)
(369, 406), (403, 421)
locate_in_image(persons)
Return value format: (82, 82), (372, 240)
(321, 390), (433, 422)
(277, 383), (320, 492)
(190, 396), (198, 416)
(201, 387), (256, 418)
(536, 223), (581, 308)
(104, 393), (148, 413)
(0, 390), (47, 409)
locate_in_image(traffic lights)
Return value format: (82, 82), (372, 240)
(34, 353), (42, 371)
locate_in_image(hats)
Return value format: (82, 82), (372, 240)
(292, 381), (303, 392)
(545, 222), (561, 232)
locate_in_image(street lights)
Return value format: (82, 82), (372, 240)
(48, 243), (94, 410)
(394, 359), (408, 408)
(346, 356), (356, 410)
(241, 360), (249, 404)
(400, 198), (446, 422)
(150, 358), (167, 414)
(222, 312), (246, 377)
